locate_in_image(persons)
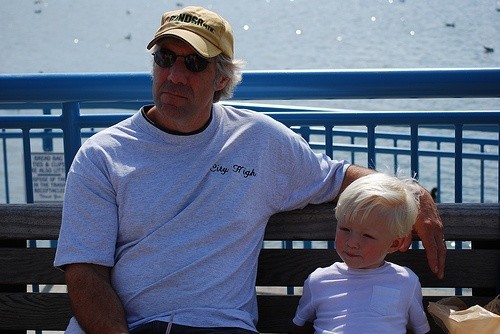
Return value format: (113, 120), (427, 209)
(53, 5), (447, 334)
(288, 172), (430, 334)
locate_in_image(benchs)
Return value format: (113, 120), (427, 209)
(0, 203), (500, 334)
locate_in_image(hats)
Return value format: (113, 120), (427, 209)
(147, 6), (235, 60)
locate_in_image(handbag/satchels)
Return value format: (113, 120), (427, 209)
(427, 301), (500, 334)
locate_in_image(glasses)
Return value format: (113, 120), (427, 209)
(152, 47), (220, 72)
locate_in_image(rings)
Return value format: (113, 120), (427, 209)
(442, 234), (446, 241)
